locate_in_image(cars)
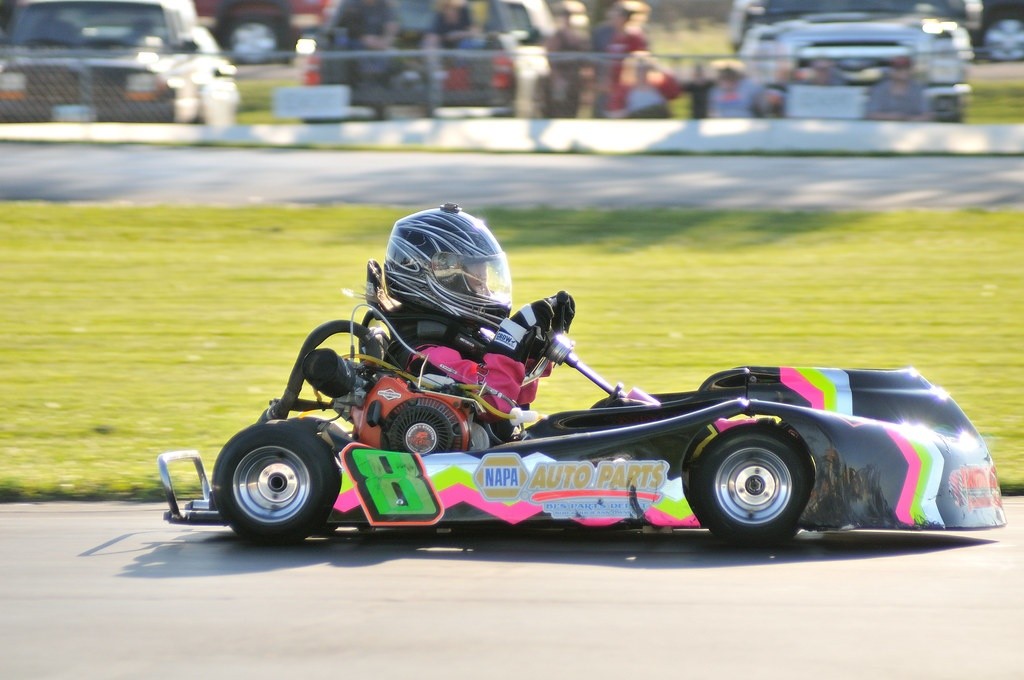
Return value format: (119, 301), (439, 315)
(723, 0), (1023, 126)
(0, 1), (240, 128)
(185, 1), (331, 67)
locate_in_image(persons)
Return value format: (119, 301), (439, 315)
(542, 1), (848, 118)
(384, 202), (575, 442)
(864, 55), (935, 122)
(348, 0), (487, 75)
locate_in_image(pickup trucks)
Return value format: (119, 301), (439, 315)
(308, 0), (561, 121)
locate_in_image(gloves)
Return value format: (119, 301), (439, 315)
(486, 298), (554, 365)
(543, 291), (576, 336)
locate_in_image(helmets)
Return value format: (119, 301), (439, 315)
(384, 202), (511, 333)
